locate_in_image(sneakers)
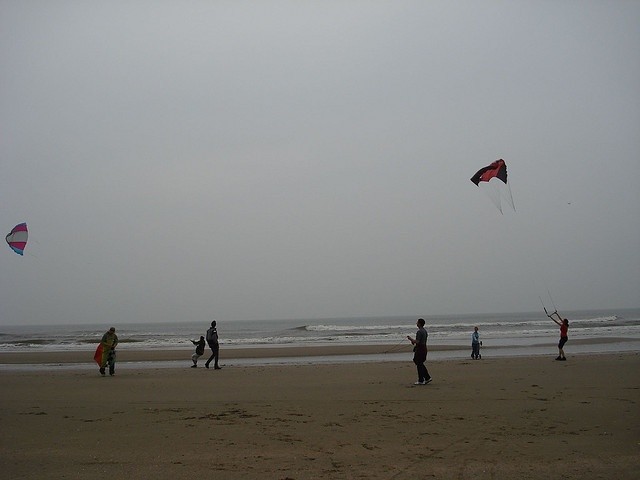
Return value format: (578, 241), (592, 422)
(414, 381), (425, 385)
(425, 378), (432, 384)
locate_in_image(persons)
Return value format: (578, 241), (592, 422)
(549, 312), (569, 361)
(205, 319), (222, 370)
(408, 318), (432, 385)
(470, 326), (480, 360)
(99, 327), (119, 376)
(190, 336), (205, 368)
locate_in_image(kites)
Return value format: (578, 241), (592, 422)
(5, 221), (28, 256)
(471, 157), (508, 187)
